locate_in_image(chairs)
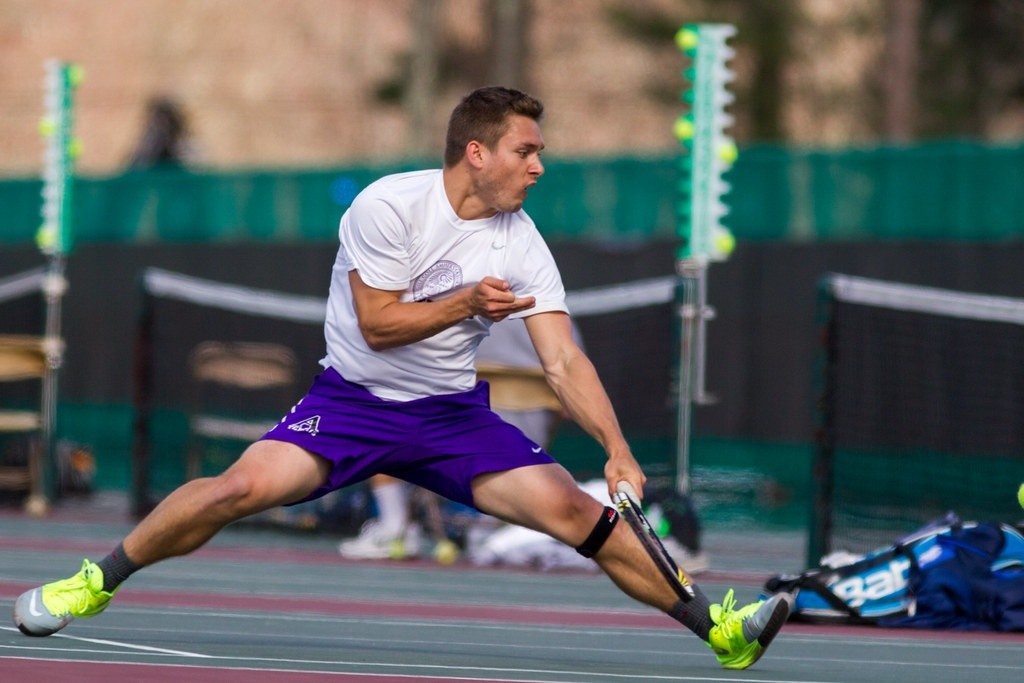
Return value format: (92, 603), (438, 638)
(390, 363), (564, 564)
(184, 344), (302, 483)
(0, 335), (48, 513)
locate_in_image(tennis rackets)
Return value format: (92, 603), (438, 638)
(611, 482), (696, 603)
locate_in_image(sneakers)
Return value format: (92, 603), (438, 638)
(701, 590), (796, 667)
(14, 558), (116, 638)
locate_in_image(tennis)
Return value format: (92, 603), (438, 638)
(434, 539), (458, 564)
(1017, 483), (1024, 510)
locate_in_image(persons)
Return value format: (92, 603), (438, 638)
(14, 87), (794, 669)
(130, 99), (187, 166)
(337, 314), (581, 558)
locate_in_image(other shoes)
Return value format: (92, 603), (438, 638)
(338, 519), (423, 559)
